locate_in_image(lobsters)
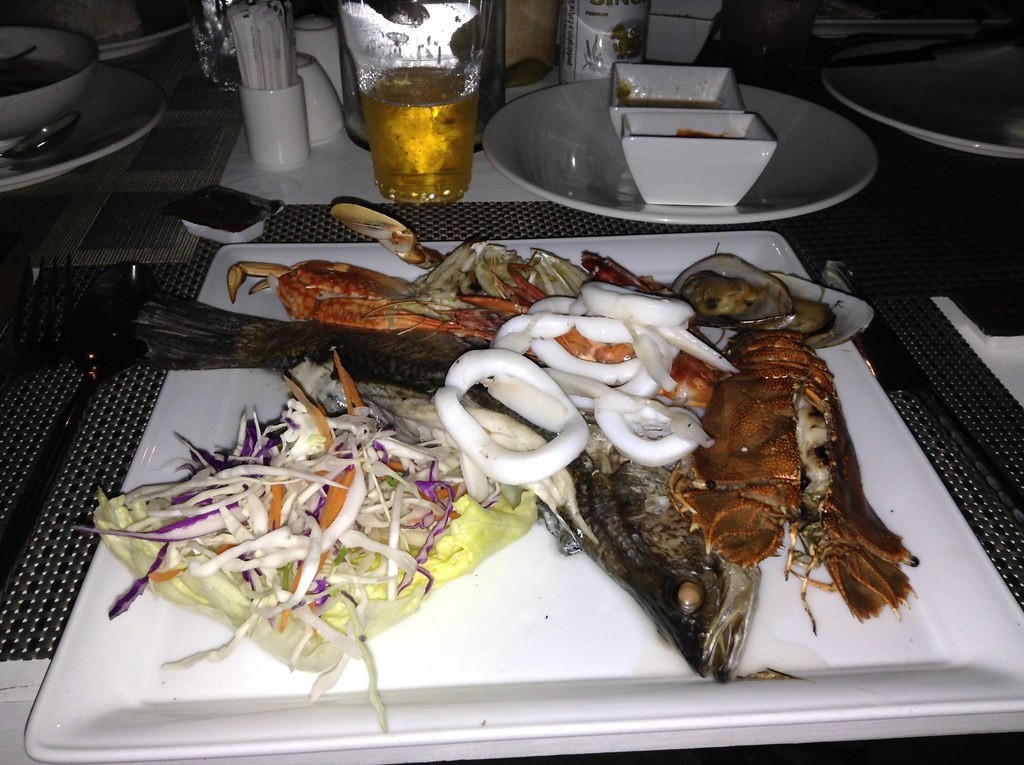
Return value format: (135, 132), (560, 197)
(665, 329), (921, 637)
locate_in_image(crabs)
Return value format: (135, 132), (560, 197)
(226, 204), (543, 362)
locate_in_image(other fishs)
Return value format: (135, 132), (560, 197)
(131, 287), (762, 684)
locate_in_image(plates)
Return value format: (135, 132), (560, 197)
(23, 229), (1024, 764)
(0, 62), (168, 194)
(480, 78), (879, 226)
(820, 44), (1024, 159)
(90, 0), (192, 61)
(0, 25), (99, 144)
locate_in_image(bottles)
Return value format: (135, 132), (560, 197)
(554, 1), (653, 89)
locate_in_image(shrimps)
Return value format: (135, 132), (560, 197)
(459, 262), (733, 409)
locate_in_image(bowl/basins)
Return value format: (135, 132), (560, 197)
(618, 110), (778, 207)
(294, 51), (344, 146)
(610, 59), (745, 144)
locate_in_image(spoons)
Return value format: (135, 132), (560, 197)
(0, 111), (82, 160)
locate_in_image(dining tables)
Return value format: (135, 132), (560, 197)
(2, 0), (1024, 765)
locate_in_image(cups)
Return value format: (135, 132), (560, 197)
(336, 0), (489, 207)
(238, 72), (311, 171)
(192, 17), (243, 92)
